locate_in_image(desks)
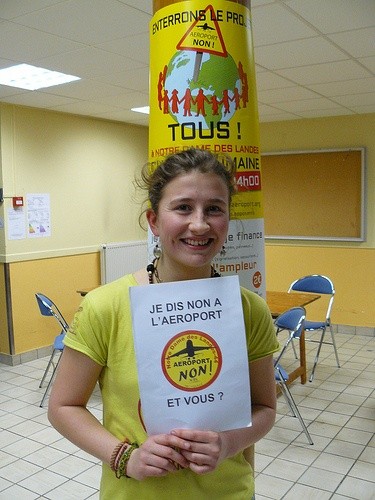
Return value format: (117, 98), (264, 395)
(266, 290), (322, 396)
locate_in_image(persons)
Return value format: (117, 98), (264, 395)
(48, 148), (280, 500)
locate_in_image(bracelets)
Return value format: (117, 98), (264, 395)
(110, 441), (139, 479)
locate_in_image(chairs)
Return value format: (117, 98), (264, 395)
(35, 293), (70, 408)
(273, 306), (314, 446)
(277, 273), (341, 382)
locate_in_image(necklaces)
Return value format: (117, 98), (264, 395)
(147, 257), (221, 284)
